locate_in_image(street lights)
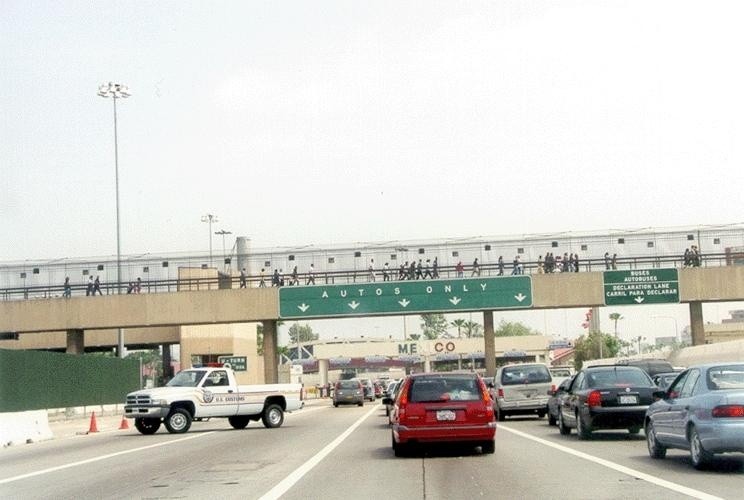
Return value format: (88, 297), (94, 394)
(197, 213), (217, 267)
(213, 225), (233, 271)
(96, 80), (132, 358)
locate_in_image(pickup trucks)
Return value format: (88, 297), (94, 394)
(121, 362), (306, 436)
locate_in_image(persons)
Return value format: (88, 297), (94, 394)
(368, 258), (378, 283)
(456, 260), (464, 278)
(92, 275), (103, 296)
(273, 264), (315, 287)
(62, 277), (72, 298)
(498, 253), (580, 276)
(86, 275), (93, 296)
(127, 281), (134, 293)
(258, 268), (266, 287)
(398, 256), (439, 279)
(684, 244), (700, 267)
(135, 278), (141, 294)
(382, 262), (391, 281)
(240, 268), (247, 288)
(471, 257), (480, 276)
(605, 253), (610, 268)
(612, 254), (618, 270)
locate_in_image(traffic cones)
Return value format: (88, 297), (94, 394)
(85, 410), (101, 433)
(117, 413), (129, 430)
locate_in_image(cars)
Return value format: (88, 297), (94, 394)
(351, 357), (687, 405)
(544, 377), (573, 427)
(556, 361), (659, 440)
(639, 361), (744, 471)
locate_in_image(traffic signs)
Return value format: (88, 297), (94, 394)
(278, 275), (533, 320)
(601, 268), (680, 305)
(216, 356), (247, 371)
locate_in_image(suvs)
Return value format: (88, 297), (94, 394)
(380, 368), (497, 459)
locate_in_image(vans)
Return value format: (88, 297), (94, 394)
(490, 361), (557, 422)
(330, 379), (364, 407)
